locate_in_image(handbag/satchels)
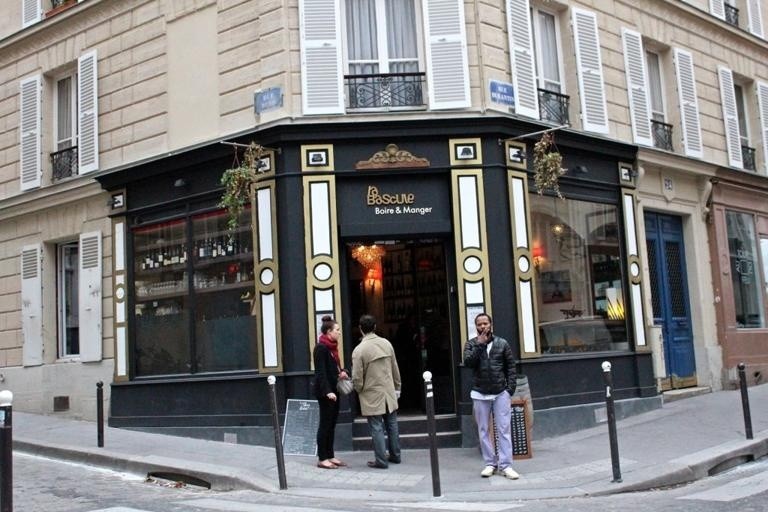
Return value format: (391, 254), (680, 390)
(336, 378), (354, 396)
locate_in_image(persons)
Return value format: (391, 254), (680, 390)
(463, 313), (521, 479)
(314, 315), (349, 469)
(352, 315), (402, 468)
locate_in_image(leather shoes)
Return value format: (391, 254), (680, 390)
(367, 461), (381, 467)
(330, 457), (346, 466)
(386, 454), (400, 463)
(318, 461), (338, 468)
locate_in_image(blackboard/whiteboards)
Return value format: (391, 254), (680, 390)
(489, 398), (532, 459)
(282, 399), (319, 457)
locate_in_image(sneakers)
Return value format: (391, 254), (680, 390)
(481, 465), (496, 477)
(501, 467), (519, 479)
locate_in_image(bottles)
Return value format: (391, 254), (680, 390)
(139, 236), (250, 297)
